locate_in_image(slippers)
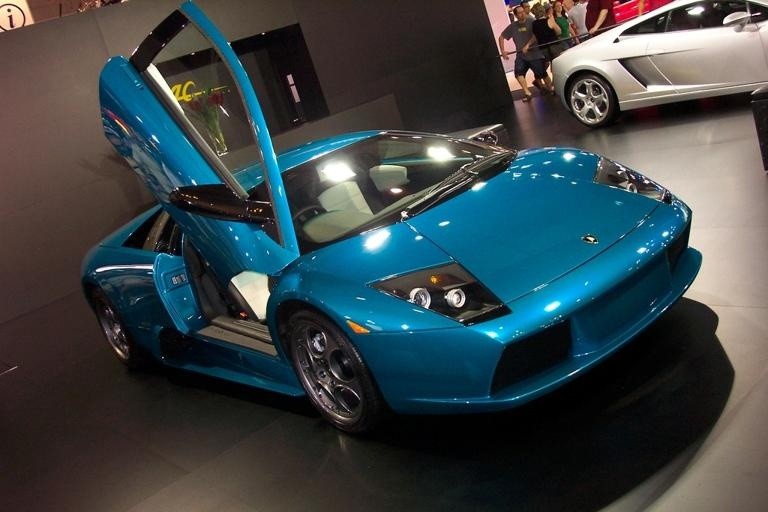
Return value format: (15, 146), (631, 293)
(522, 95), (532, 102)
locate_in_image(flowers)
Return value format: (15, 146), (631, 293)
(180, 75), (233, 152)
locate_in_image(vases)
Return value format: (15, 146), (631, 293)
(197, 109), (228, 157)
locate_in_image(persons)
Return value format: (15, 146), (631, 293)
(498, 0), (618, 103)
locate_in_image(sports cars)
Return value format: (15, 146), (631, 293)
(552, 0), (767, 129)
(80, 0), (703, 439)
